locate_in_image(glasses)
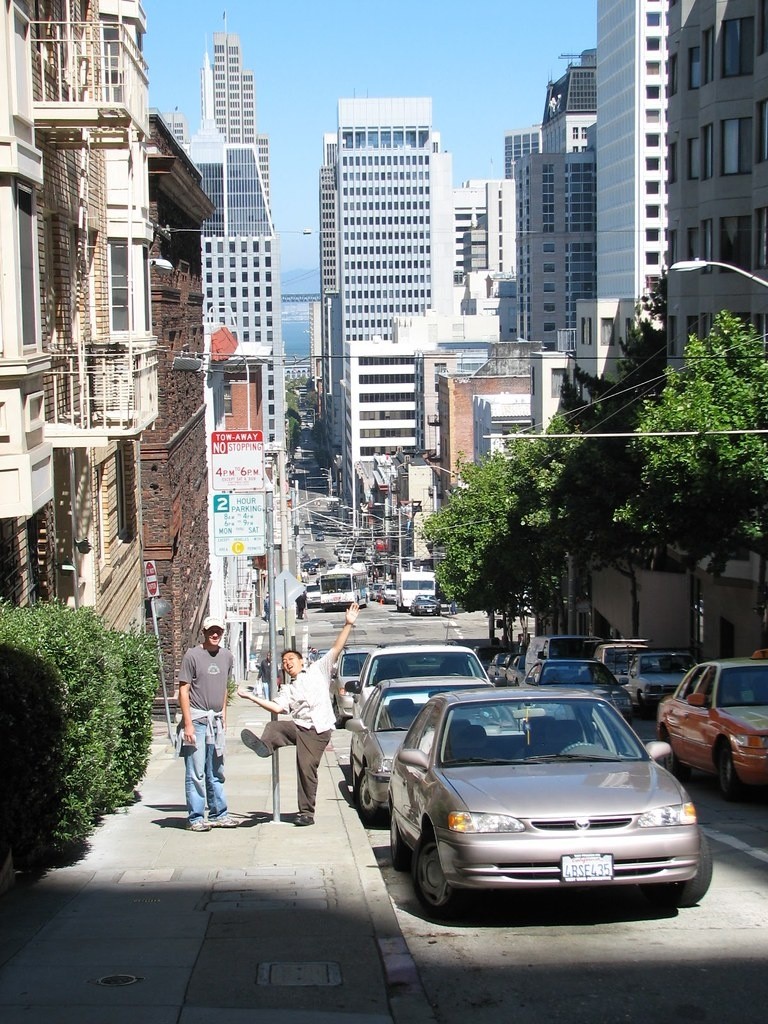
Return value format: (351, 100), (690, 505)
(206, 629), (222, 636)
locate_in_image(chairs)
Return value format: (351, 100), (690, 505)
(389, 698), (415, 729)
(546, 718), (583, 755)
(440, 662), (468, 675)
(344, 660), (363, 674)
(458, 725), (486, 757)
(376, 661), (403, 678)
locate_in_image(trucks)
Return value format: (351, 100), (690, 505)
(396, 571), (437, 613)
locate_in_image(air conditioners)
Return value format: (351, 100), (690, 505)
(95, 334), (158, 418)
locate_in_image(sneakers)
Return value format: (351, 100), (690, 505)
(209, 816), (238, 827)
(185, 819), (211, 831)
(294, 815), (314, 825)
(241, 729), (270, 757)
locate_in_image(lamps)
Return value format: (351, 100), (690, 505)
(148, 256), (173, 270)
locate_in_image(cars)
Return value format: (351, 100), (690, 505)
(599, 653), (702, 720)
(410, 595), (441, 616)
(473, 646), (525, 687)
(387, 685), (712, 920)
(521, 657), (634, 728)
(657, 648), (768, 802)
(345, 677), (521, 825)
(369, 577), (397, 605)
(301, 521), (373, 575)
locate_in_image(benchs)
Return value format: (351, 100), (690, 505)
(450, 716), (555, 755)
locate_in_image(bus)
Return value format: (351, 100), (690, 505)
(320, 563), (369, 611)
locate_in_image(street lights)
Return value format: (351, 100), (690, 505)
(288, 498), (338, 549)
(363, 513), (385, 532)
(374, 502), (411, 571)
(320, 467), (332, 496)
(343, 506), (364, 528)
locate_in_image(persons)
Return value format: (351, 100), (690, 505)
(175, 617), (239, 831)
(238, 603), (361, 825)
(264, 594), (269, 622)
(308, 645), (317, 667)
(296, 591), (307, 619)
(257, 651), (270, 701)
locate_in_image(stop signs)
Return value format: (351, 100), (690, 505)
(142, 562), (161, 598)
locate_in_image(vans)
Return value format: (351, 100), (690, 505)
(525, 635), (651, 680)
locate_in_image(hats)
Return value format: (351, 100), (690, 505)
(203, 617), (224, 630)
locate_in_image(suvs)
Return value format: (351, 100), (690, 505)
(305, 584), (321, 609)
(319, 642), (507, 718)
